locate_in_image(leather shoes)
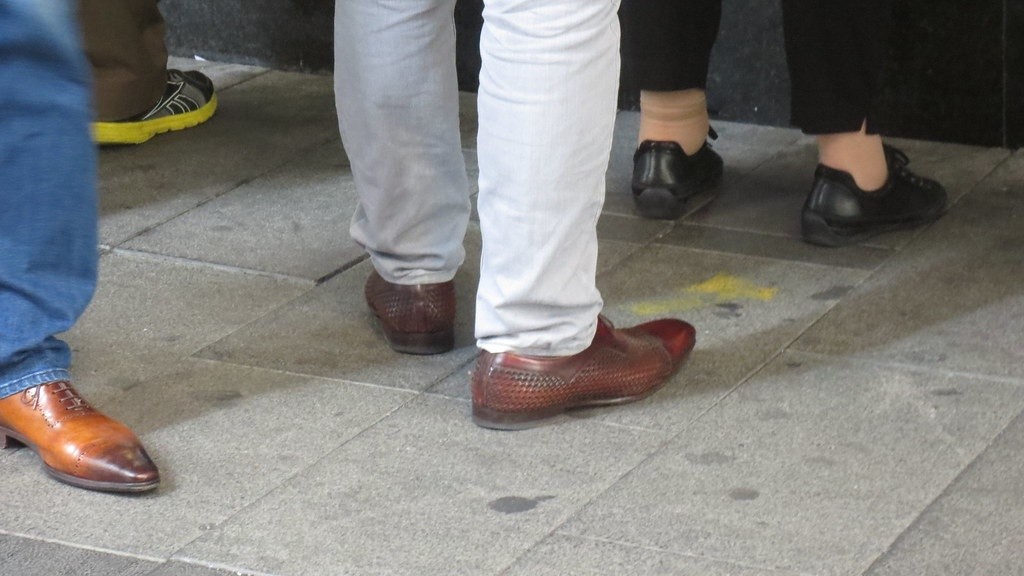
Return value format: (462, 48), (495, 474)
(630, 124), (723, 219)
(800, 142), (949, 245)
(470, 313), (696, 430)
(0, 380), (161, 492)
(365, 267), (455, 354)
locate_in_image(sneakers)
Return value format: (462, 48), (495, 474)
(92, 69), (218, 143)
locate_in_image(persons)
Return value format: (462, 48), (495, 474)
(0, 0), (160, 495)
(330, 1), (698, 431)
(618, 1), (948, 247)
(71, 1), (218, 145)
